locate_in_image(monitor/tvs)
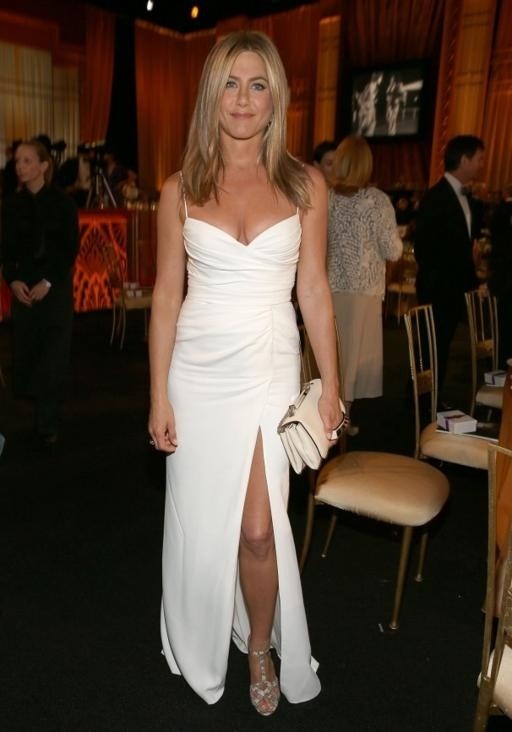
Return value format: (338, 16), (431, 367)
(341, 66), (433, 143)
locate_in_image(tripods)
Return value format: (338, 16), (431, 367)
(86, 165), (118, 209)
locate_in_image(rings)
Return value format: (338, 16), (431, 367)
(150, 440), (157, 446)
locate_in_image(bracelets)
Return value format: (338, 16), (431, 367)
(43, 278), (52, 288)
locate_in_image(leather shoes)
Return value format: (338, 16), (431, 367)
(245, 636), (282, 716)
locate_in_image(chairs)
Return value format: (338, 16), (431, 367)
(404, 304), (500, 470)
(384, 251), (417, 326)
(296, 316), (449, 632)
(474, 444), (512, 732)
(98, 242), (152, 349)
(465, 293), (512, 432)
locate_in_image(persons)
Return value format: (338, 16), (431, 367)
(487, 185), (511, 375)
(47, 137), (143, 210)
(385, 72), (407, 136)
(470, 182), (488, 240)
(354, 70), (382, 137)
(149, 30), (344, 719)
(328, 134), (403, 455)
(406, 135), (485, 417)
(0, 141), (79, 447)
(314, 140), (338, 185)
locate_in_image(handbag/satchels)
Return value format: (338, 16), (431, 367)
(277, 377), (353, 475)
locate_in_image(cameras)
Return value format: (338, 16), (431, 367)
(77, 138), (111, 165)
